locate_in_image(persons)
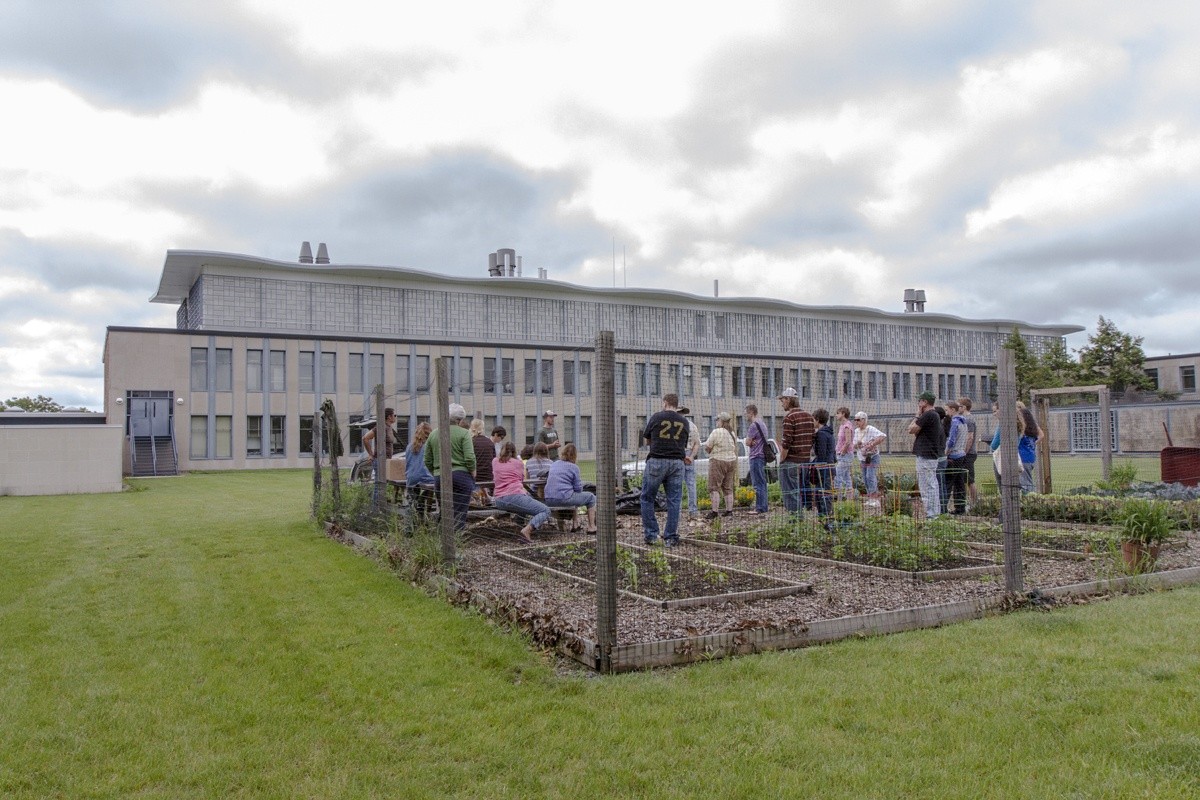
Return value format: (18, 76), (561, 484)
(990, 400), (1045, 526)
(677, 405), (768, 522)
(908, 391), (977, 522)
(363, 402), (596, 545)
(776, 386), (888, 534)
(640, 393), (690, 544)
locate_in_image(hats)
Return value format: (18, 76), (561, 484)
(775, 387), (799, 399)
(544, 410), (557, 419)
(677, 407), (690, 415)
(715, 412), (731, 422)
(915, 391), (936, 401)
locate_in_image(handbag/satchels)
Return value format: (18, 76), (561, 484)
(993, 444), (1025, 476)
(763, 439), (779, 462)
(859, 425), (879, 456)
(809, 459), (823, 485)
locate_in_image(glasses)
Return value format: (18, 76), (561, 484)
(853, 418), (863, 421)
(391, 414), (397, 418)
(500, 435), (504, 441)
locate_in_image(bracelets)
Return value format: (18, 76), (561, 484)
(915, 416), (919, 420)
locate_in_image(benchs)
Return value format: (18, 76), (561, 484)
(431, 506), (578, 538)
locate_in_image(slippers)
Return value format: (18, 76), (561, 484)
(517, 535), (535, 545)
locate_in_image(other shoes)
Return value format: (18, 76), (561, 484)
(587, 529), (597, 535)
(863, 498), (876, 506)
(705, 511), (718, 519)
(664, 538), (677, 547)
(747, 511), (766, 517)
(646, 536), (659, 545)
(722, 510), (733, 517)
(571, 525), (584, 533)
(686, 515), (698, 522)
(869, 499), (881, 508)
(949, 509), (967, 515)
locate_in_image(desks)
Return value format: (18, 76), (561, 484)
(417, 478), (546, 511)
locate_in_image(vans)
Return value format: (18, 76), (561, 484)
(621, 437), (780, 488)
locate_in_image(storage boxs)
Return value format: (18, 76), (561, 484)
(386, 458), (407, 481)
